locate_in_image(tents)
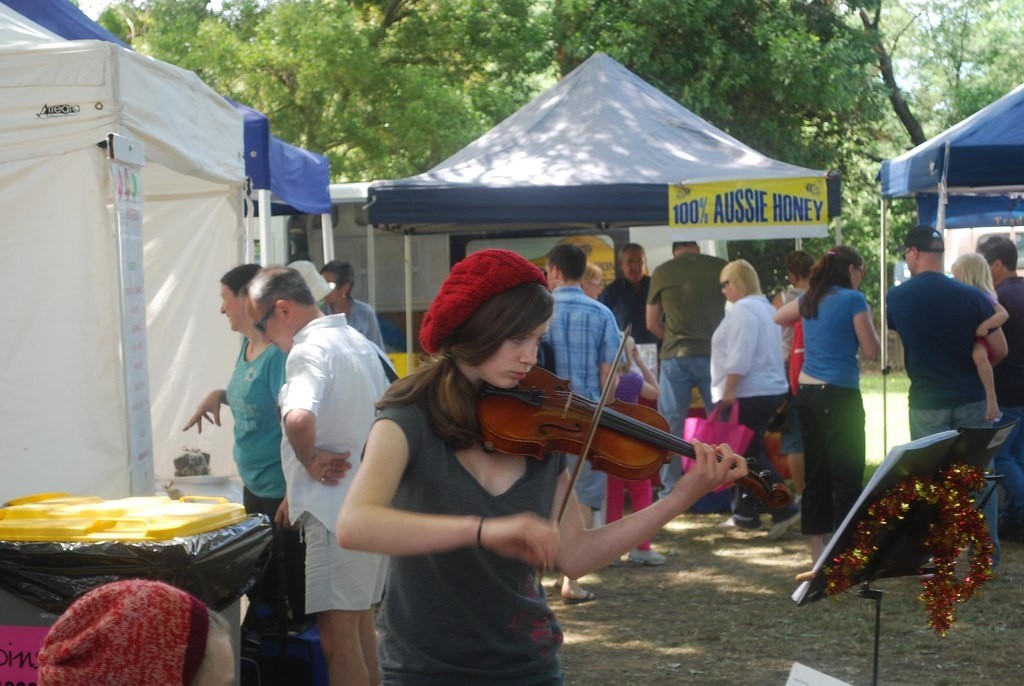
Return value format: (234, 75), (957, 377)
(0, 0), (1024, 509)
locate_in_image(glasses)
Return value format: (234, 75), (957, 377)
(902, 248), (911, 259)
(854, 266), (866, 278)
(252, 295), (287, 333)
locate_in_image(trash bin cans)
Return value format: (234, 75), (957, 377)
(0, 492), (275, 686)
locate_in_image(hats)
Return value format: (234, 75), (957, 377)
(35, 578), (209, 686)
(287, 259), (336, 302)
(418, 248), (552, 357)
(893, 225), (945, 254)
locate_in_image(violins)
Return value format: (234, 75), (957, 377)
(473, 364), (793, 509)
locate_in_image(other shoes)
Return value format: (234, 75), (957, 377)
(608, 557), (622, 567)
(767, 509), (801, 541)
(628, 547), (666, 565)
(719, 518), (763, 531)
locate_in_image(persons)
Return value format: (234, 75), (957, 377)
(40, 222), (1024, 686)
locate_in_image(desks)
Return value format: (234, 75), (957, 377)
(155, 479), (243, 504)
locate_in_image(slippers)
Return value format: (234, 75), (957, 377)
(563, 590), (599, 604)
(984, 410), (1003, 421)
(553, 582), (562, 591)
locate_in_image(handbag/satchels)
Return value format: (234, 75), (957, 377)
(240, 527), (330, 685)
(682, 397), (756, 494)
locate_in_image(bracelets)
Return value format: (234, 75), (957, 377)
(296, 448), (317, 465)
(477, 516), (488, 548)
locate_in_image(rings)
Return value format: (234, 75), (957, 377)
(320, 477), (327, 482)
